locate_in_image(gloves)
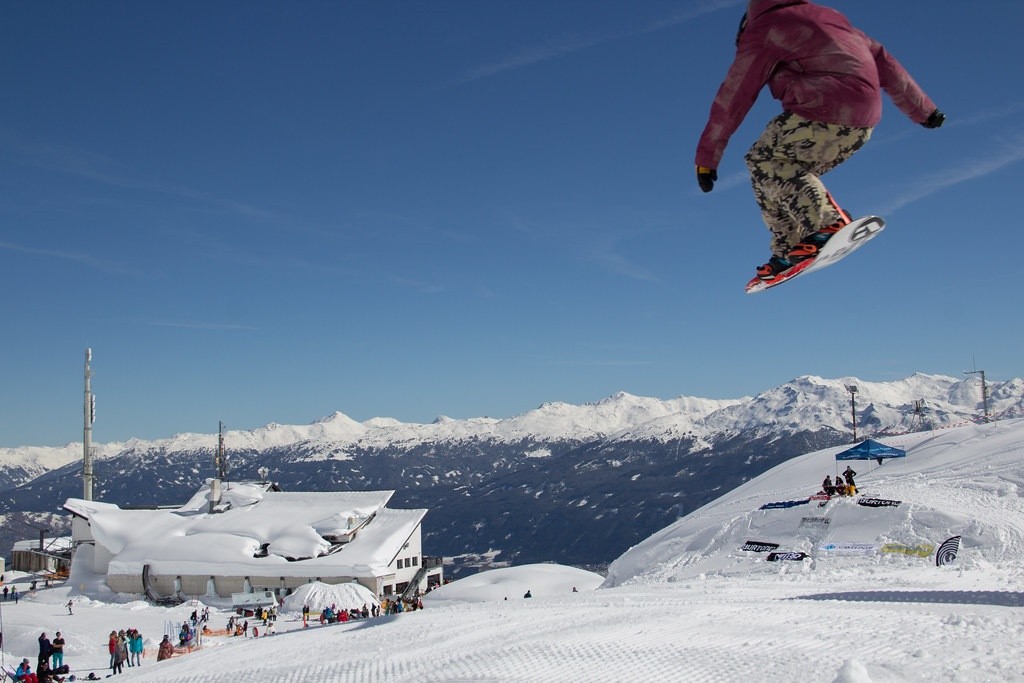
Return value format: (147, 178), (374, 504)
(919, 108), (946, 128)
(696, 165), (717, 192)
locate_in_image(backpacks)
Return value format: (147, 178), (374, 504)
(181, 636), (185, 640)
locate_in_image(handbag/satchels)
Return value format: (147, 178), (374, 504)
(58, 664), (69, 674)
(122, 654), (125, 660)
(47, 647), (54, 657)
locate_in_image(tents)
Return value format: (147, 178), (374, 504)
(836, 439), (907, 478)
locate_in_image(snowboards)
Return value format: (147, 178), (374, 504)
(745, 215), (886, 296)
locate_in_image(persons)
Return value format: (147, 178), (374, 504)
(15, 632), (65, 683)
(524, 589), (531, 597)
(157, 635), (174, 661)
(227, 607), (247, 637)
(695, 0), (945, 285)
(385, 594), (423, 615)
(179, 607), (211, 645)
(109, 629), (143, 674)
(427, 580), (448, 593)
(573, 587), (578, 592)
(843, 466), (859, 493)
(303, 603), (380, 624)
(279, 587), (292, 607)
(257, 605), (276, 626)
(836, 476), (846, 495)
(823, 475), (835, 495)
(1, 575), (49, 604)
(65, 600), (73, 614)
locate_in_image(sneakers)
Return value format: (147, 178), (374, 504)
(788, 210), (852, 262)
(757, 254), (796, 279)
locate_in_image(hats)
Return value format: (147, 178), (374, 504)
(121, 629), (126, 634)
(23, 658), (29, 663)
(736, 12), (747, 45)
(133, 629), (138, 634)
